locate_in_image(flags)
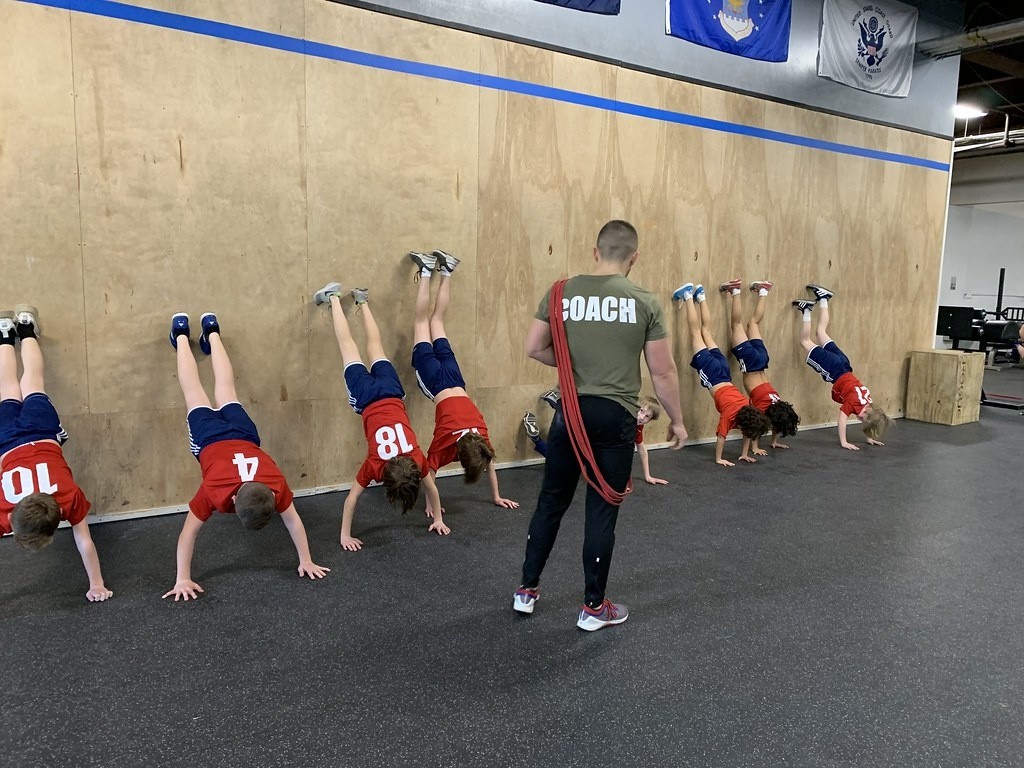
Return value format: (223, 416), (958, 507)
(817, 0), (918, 97)
(666, 0), (792, 63)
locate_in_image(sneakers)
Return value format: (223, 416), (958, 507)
(169, 312), (190, 351)
(0, 310), (19, 339)
(14, 304), (41, 341)
(749, 280), (773, 295)
(432, 249), (461, 272)
(350, 287), (369, 315)
(314, 282), (343, 311)
(540, 389), (561, 409)
(806, 284), (835, 304)
(513, 587), (540, 614)
(409, 250), (438, 284)
(672, 282), (694, 309)
(792, 298), (816, 315)
(692, 283), (704, 303)
(719, 278), (742, 294)
(198, 312), (220, 355)
(522, 412), (540, 442)
(576, 598), (629, 632)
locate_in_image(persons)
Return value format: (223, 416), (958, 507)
(792, 284), (888, 450)
(672, 282), (770, 466)
(162, 312), (330, 601)
(1016, 324), (1024, 359)
(522, 391), (669, 484)
(410, 250), (519, 518)
(313, 282), (451, 551)
(513, 219), (688, 631)
(0, 304), (112, 602)
(719, 278), (801, 456)
